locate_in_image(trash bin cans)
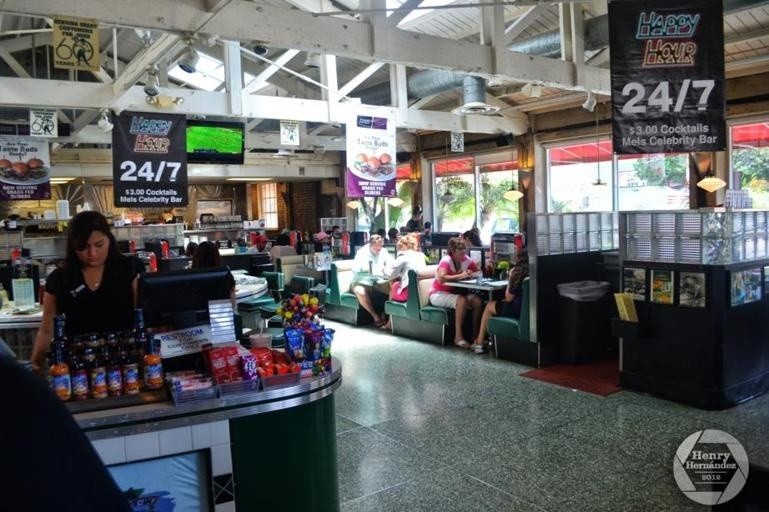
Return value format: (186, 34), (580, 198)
(557, 279), (609, 364)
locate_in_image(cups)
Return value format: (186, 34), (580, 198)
(193, 215), (266, 230)
(56, 200), (69, 219)
(44, 210), (56, 220)
(248, 333), (272, 349)
(8, 220), (17, 229)
(303, 255), (318, 268)
(476, 272), (483, 286)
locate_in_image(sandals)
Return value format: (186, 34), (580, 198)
(453, 337), (468, 349)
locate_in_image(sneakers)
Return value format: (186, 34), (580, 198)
(471, 340), (484, 353)
(375, 318), (391, 330)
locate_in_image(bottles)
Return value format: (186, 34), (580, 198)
(322, 243), (331, 256)
(38, 285), (45, 305)
(43, 308), (165, 401)
(0, 284), (10, 307)
(105, 211), (145, 226)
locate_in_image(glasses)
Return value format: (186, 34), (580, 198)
(376, 235), (384, 239)
(455, 246), (466, 250)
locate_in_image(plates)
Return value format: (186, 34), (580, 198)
(0, 168), (49, 186)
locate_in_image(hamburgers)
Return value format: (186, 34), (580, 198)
(0, 159), (47, 179)
(354, 153), (393, 177)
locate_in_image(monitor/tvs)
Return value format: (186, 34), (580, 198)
(137, 268), (229, 329)
(186, 119), (245, 164)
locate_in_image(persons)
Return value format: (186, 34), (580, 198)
(350, 205), (432, 329)
(187, 241), (235, 308)
(429, 227), (528, 354)
(32, 210), (140, 373)
(237, 225), (341, 271)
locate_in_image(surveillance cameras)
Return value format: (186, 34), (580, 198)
(254, 45), (268, 56)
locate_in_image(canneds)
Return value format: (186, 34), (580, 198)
(50, 307), (154, 401)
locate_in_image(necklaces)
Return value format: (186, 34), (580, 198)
(94, 266), (103, 287)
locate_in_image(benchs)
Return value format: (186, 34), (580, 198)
(261, 254), (530, 364)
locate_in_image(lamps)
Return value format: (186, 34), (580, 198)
(696, 150), (726, 193)
(439, 134), (456, 204)
(98, 35), (321, 132)
(502, 135), (524, 201)
